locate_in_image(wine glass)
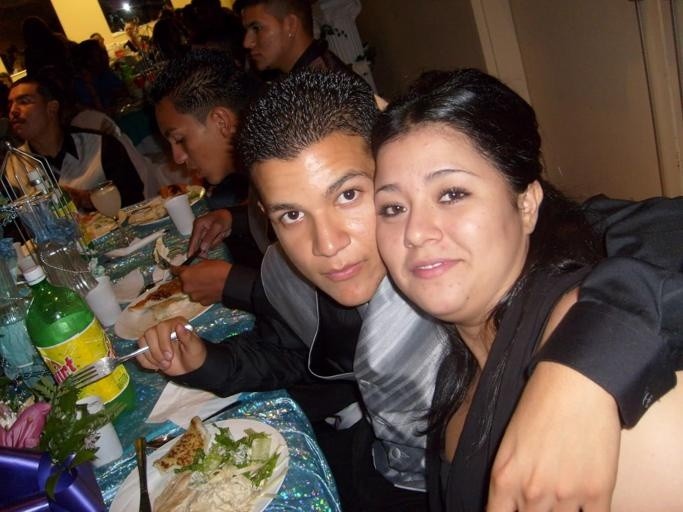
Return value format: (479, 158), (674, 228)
(90, 180), (136, 249)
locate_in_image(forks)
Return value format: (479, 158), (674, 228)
(158, 250), (179, 273)
(59, 325), (193, 395)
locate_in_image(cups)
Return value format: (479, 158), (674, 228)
(84, 274), (122, 326)
(81, 400), (122, 467)
(163, 193), (195, 235)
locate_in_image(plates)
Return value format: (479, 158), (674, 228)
(115, 282), (214, 340)
(129, 184), (207, 241)
(81, 209), (128, 242)
(108, 420), (291, 512)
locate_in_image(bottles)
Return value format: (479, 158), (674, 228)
(29, 168), (95, 252)
(22, 264), (136, 418)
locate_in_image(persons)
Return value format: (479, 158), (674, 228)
(1, 0), (376, 324)
(368, 68), (682, 512)
(134, 65), (682, 511)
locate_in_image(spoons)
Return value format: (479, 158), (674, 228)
(147, 400), (242, 448)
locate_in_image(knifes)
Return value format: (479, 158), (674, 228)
(134, 437), (152, 511)
